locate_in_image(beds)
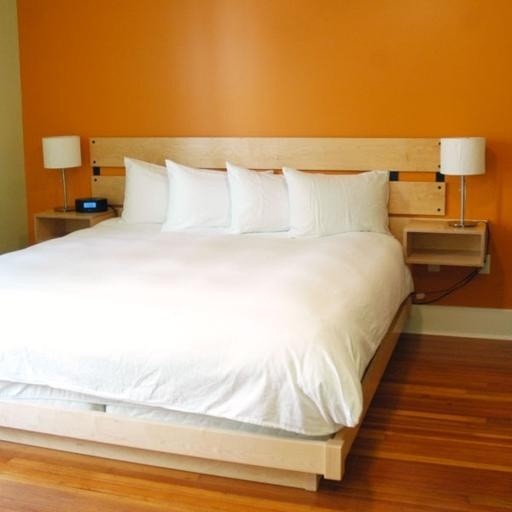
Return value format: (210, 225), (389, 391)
(0, 134), (447, 498)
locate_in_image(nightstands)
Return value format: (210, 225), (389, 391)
(33, 207), (116, 244)
(401, 219), (488, 267)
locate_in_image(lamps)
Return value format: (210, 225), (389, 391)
(41, 135), (82, 213)
(439, 137), (486, 229)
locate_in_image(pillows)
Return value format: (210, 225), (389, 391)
(117, 154), (392, 241)
